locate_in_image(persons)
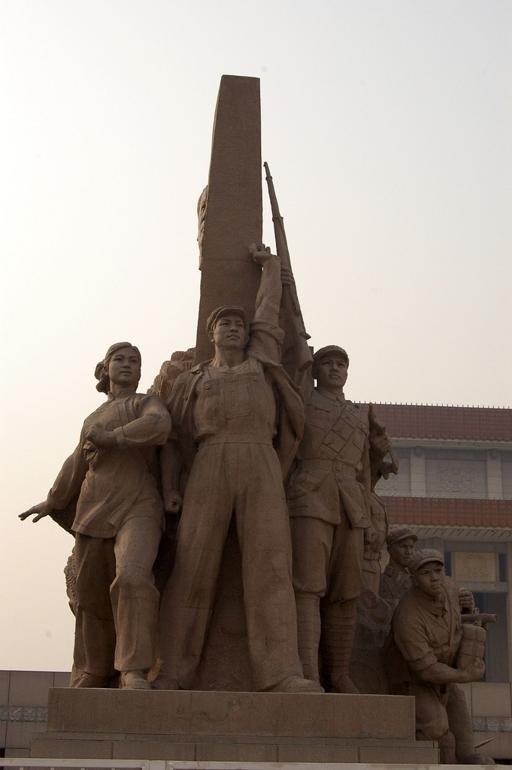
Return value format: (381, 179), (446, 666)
(156, 236), (328, 693)
(281, 290), (393, 694)
(391, 547), (493, 765)
(15, 339), (174, 687)
(374, 523), (424, 620)
